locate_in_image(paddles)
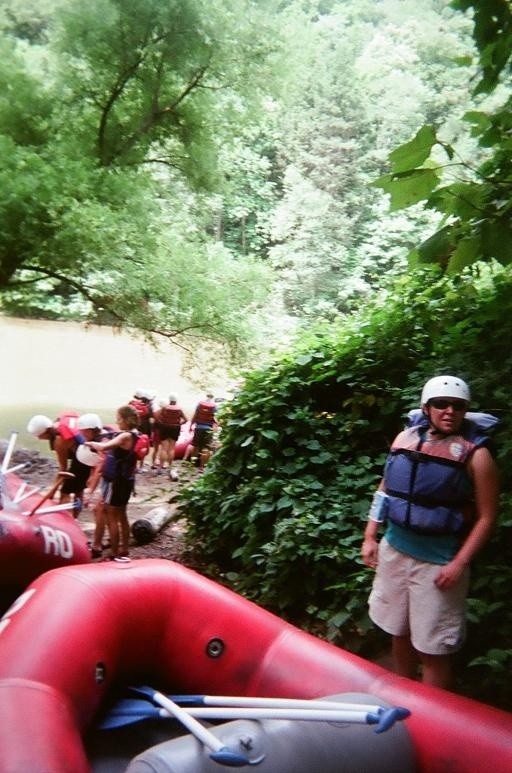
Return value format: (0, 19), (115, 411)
(0, 430), (82, 520)
(100, 697), (400, 733)
(139, 692), (411, 722)
(122, 681), (250, 768)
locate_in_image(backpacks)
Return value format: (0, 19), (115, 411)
(131, 431), (151, 461)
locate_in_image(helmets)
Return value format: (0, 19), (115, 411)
(26, 414), (56, 434)
(205, 387), (215, 397)
(419, 373), (474, 410)
(74, 442), (105, 467)
(77, 412), (103, 431)
(167, 392), (178, 401)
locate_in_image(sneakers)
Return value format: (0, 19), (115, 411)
(85, 537), (131, 563)
(135, 466), (149, 474)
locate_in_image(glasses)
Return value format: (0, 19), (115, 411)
(427, 398), (467, 411)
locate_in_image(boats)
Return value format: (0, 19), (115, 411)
(174, 418), (220, 459)
(1, 463), (93, 589)
(1, 557), (511, 772)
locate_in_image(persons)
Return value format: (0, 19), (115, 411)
(181, 394), (217, 461)
(28, 386), (188, 558)
(360, 376), (500, 690)
